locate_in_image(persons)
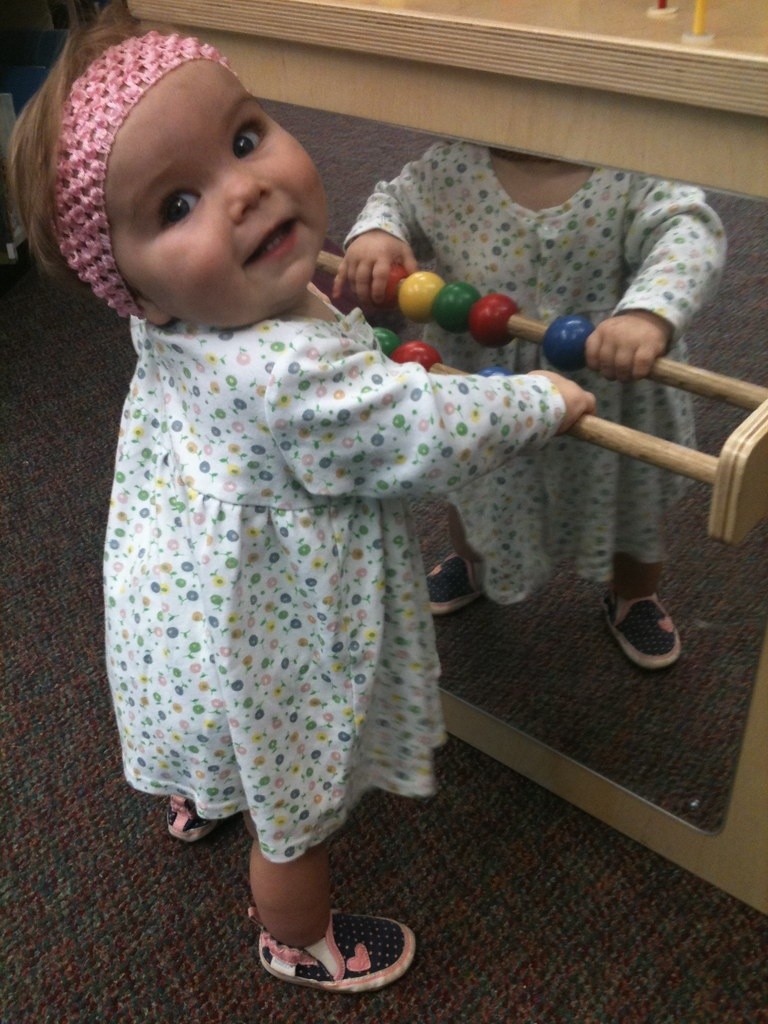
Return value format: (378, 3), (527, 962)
(331, 134), (726, 668)
(10, 0), (599, 991)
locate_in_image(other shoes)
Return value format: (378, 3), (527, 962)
(426, 552), (485, 614)
(246, 901), (415, 991)
(166, 793), (219, 842)
(601, 589), (681, 669)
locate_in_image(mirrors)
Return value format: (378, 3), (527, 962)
(138, 19), (768, 916)
